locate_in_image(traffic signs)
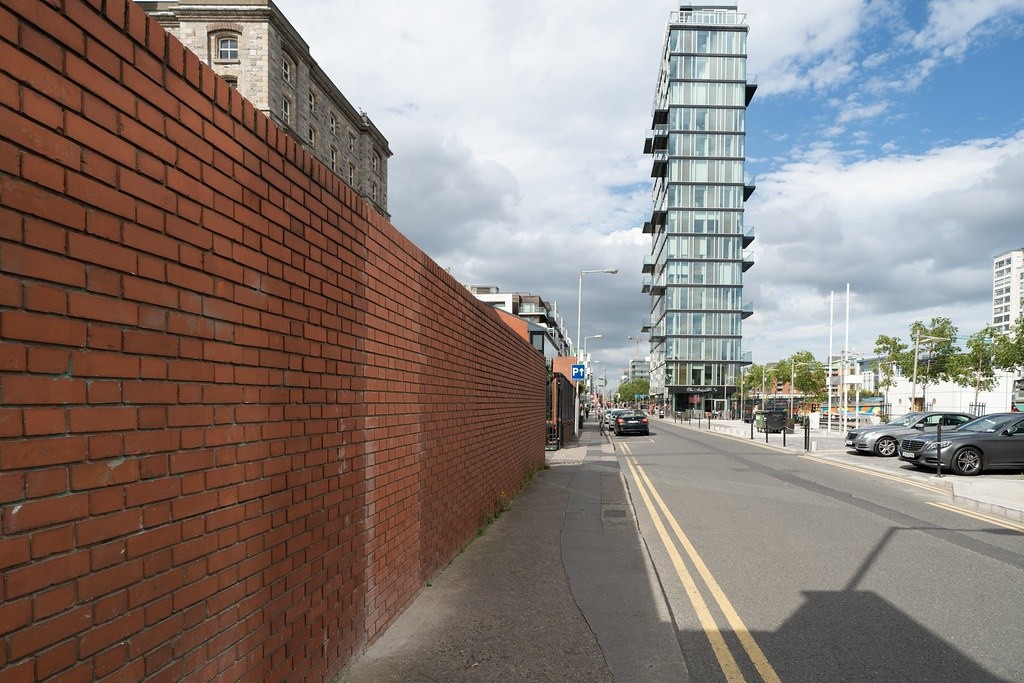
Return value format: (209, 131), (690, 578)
(570, 364), (586, 381)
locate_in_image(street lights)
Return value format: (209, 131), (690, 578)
(573, 268), (619, 440)
(589, 360), (600, 405)
(739, 367), (754, 421)
(908, 329), (951, 414)
(724, 374), (738, 420)
(583, 334), (603, 422)
(761, 364), (780, 410)
(790, 358), (811, 419)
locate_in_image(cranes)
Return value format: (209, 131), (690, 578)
(625, 335), (649, 359)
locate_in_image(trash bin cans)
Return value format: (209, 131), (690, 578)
(793, 414), (798, 423)
(754, 410), (784, 433)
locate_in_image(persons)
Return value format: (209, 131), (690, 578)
(597, 404), (600, 419)
(585, 405), (589, 419)
(580, 406), (584, 415)
(650, 402), (653, 415)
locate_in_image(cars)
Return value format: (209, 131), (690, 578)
(897, 411), (1024, 476)
(612, 409), (650, 436)
(603, 408), (629, 430)
(843, 410), (998, 458)
